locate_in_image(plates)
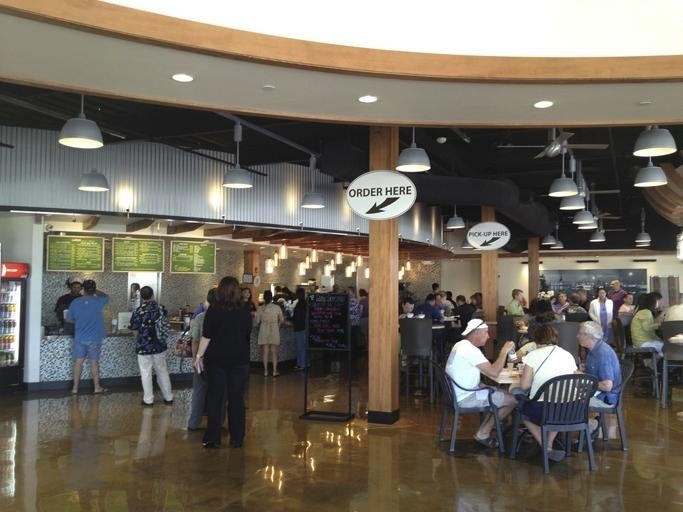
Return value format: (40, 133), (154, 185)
(499, 372), (512, 378)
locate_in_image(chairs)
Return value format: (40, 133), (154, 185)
(560, 357), (635, 453)
(399, 301), (682, 411)
(509, 373), (598, 473)
(430, 361), (506, 456)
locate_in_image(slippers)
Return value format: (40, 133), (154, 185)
(93, 388), (113, 394)
(473, 434), (501, 449)
(588, 416), (601, 442)
(68, 390), (80, 395)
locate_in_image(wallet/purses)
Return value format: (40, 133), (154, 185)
(193, 360), (207, 377)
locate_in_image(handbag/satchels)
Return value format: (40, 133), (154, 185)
(173, 336), (192, 357)
(155, 315), (171, 342)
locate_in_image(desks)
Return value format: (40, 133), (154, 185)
(479, 367), (576, 460)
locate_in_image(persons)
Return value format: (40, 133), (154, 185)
(503, 287), (590, 342)
(126, 281), (141, 309)
(398, 295), (419, 320)
(64, 280), (111, 397)
(182, 277), (367, 454)
(577, 320), (623, 450)
(589, 281), (665, 372)
(127, 285), (175, 409)
(444, 319), (517, 449)
(421, 282), (482, 344)
(54, 274), (82, 328)
(67, 394), (248, 511)
(505, 321), (580, 462)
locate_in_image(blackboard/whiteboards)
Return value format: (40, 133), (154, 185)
(305, 293), (352, 351)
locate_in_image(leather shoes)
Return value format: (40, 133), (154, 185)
(141, 401), (153, 406)
(164, 400), (173, 404)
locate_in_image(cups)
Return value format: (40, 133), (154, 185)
(507, 347), (518, 361)
(508, 363), (513, 372)
(407, 313), (414, 318)
(110, 319), (117, 333)
(518, 365), (524, 378)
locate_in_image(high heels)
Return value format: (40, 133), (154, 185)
(202, 439), (221, 448)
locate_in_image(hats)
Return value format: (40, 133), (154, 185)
(462, 318), (488, 336)
(69, 277), (84, 285)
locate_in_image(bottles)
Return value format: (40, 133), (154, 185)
(178, 304), (189, 317)
(0, 336), (15, 350)
(0, 320), (17, 333)
(1, 304), (17, 318)
(0, 351), (16, 366)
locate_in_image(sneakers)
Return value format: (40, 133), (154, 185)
(546, 449), (566, 461)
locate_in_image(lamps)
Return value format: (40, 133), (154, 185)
(301, 192), (325, 209)
(222, 168), (254, 189)
(559, 188), (586, 212)
(632, 129), (676, 157)
(548, 178), (579, 199)
(58, 118), (104, 150)
(541, 235), (564, 248)
(633, 166), (667, 188)
(447, 217), (465, 230)
(572, 210), (598, 230)
(394, 147), (431, 172)
(77, 168), (109, 191)
(589, 232), (607, 243)
(634, 232), (652, 248)
(264, 241), (412, 281)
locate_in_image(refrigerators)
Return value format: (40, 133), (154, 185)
(1, 260), (29, 389)
